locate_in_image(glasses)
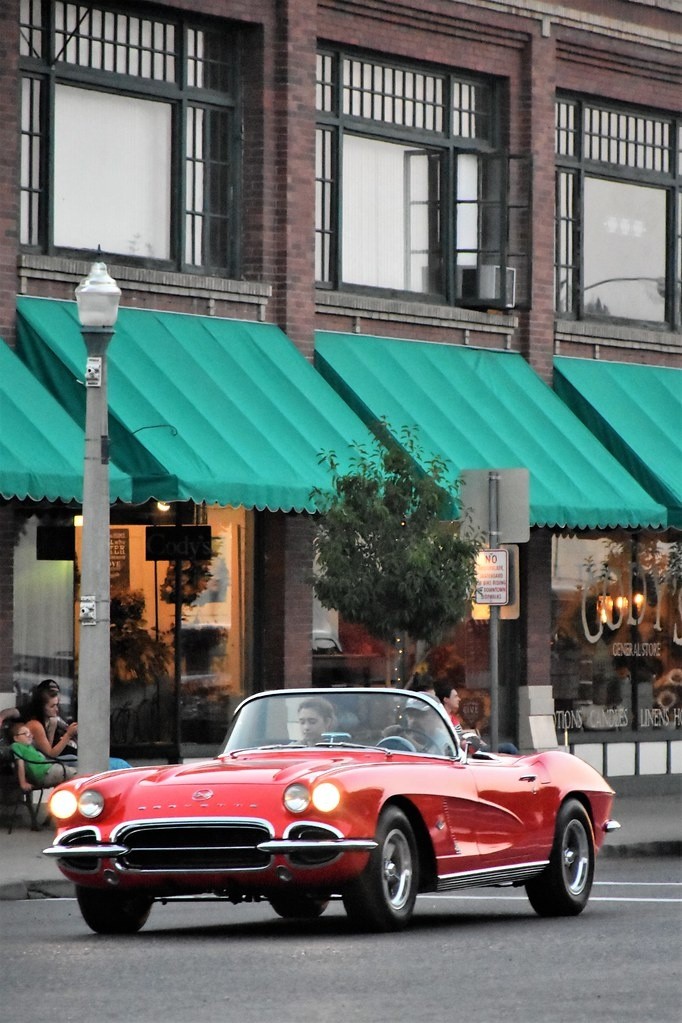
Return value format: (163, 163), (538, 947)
(16, 733), (33, 737)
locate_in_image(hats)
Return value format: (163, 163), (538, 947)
(37, 680), (59, 692)
(402, 692), (440, 714)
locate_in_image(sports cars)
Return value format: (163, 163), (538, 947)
(42, 686), (621, 931)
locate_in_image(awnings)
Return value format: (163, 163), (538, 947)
(0, 338), (132, 505)
(313, 330), (668, 529)
(16, 297), (420, 517)
(552, 356), (682, 534)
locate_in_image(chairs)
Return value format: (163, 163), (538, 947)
(8, 749), (77, 835)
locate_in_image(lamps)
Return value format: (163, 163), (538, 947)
(595, 592), (614, 624)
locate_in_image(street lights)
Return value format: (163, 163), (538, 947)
(73, 258), (125, 781)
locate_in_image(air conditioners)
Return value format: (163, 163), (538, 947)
(456, 264), (516, 308)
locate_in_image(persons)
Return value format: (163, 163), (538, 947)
(0, 679), (133, 791)
(417, 683), (519, 755)
(291, 697), (334, 746)
(382, 691), (472, 759)
